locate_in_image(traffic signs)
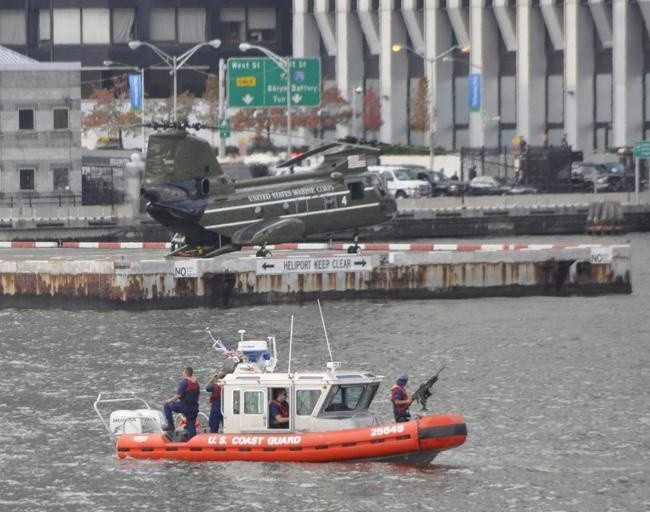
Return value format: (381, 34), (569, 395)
(225, 56), (323, 110)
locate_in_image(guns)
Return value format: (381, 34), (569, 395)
(403, 367), (445, 411)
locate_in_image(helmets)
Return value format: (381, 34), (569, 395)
(396, 375), (408, 385)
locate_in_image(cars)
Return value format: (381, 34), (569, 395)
(555, 162), (642, 192)
(409, 169), (498, 195)
(368, 167), (432, 199)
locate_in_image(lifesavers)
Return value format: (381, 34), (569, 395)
(179, 419), (202, 429)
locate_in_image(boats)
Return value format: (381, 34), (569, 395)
(94, 298), (468, 464)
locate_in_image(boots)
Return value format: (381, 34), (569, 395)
(161, 419), (175, 431)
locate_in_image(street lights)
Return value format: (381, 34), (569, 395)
(239, 43), (296, 166)
(126, 39), (223, 124)
(102, 59), (171, 126)
(391, 43), (483, 174)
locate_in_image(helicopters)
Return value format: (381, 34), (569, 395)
(142, 131), (398, 256)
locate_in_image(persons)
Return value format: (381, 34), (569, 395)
(391, 375), (412, 422)
(468, 166), (477, 180)
(162, 367), (200, 441)
(450, 171), (458, 180)
(270, 388), (289, 428)
(205, 371), (225, 433)
